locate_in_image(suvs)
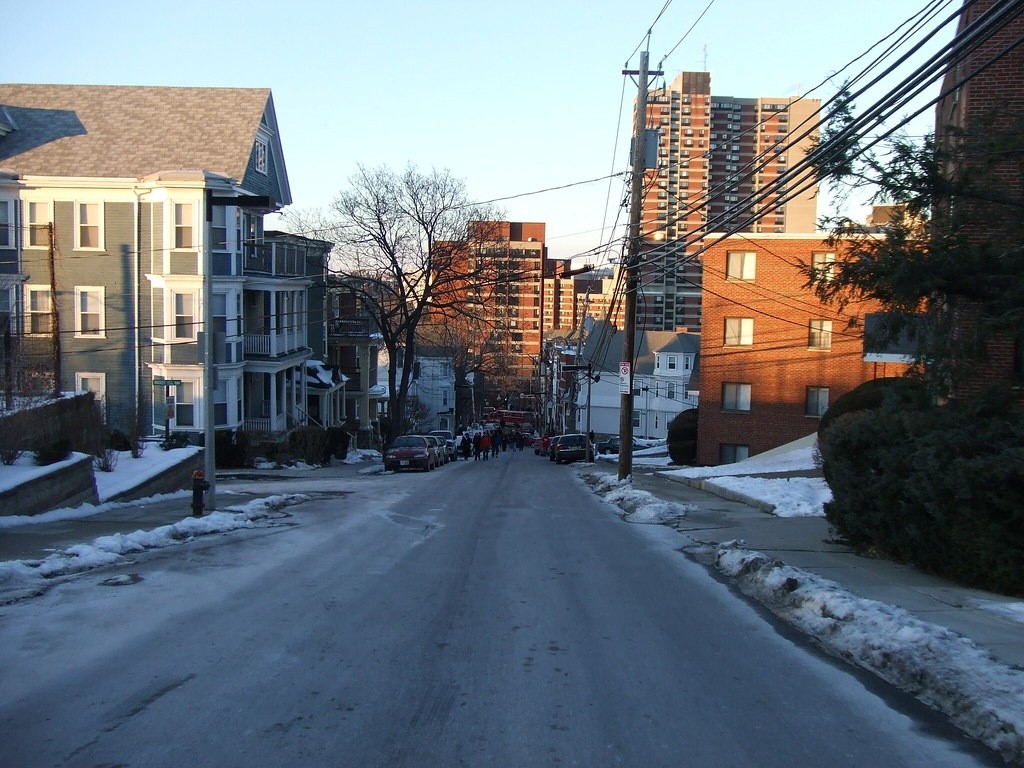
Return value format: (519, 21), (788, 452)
(427, 430), (458, 462)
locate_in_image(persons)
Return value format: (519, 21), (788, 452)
(461, 419), (551, 461)
(590, 430), (595, 443)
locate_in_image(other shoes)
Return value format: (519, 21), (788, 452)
(465, 457), (489, 461)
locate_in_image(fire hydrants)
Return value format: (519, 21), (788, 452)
(189, 469), (211, 516)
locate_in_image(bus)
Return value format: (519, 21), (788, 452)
(490, 410), (532, 429)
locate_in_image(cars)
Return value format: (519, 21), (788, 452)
(434, 435), (450, 464)
(597, 436), (619, 455)
(383, 434), (436, 472)
(554, 433), (594, 464)
(547, 435), (560, 461)
(454, 419), (504, 457)
(516, 422), (555, 457)
(422, 435), (442, 467)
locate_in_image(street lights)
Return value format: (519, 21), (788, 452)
(196, 191), (272, 511)
(520, 394), (542, 438)
(564, 364), (592, 463)
